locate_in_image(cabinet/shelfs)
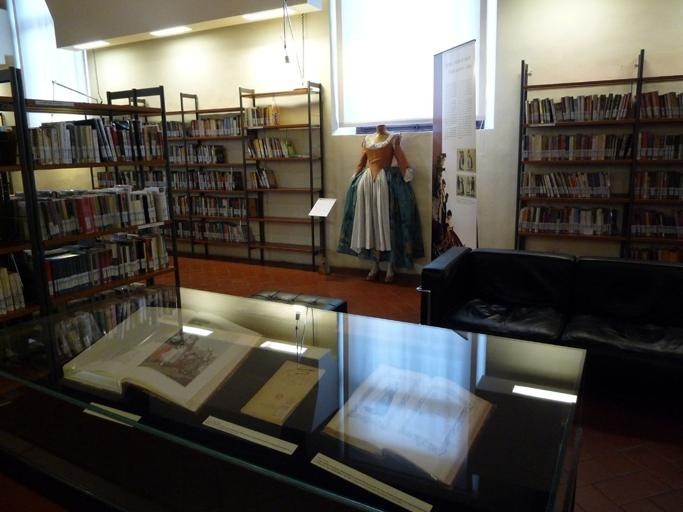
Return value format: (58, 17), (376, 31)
(87, 81), (326, 272)
(0, 66), (184, 397)
(514, 48), (683, 263)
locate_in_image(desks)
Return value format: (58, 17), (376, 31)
(0, 283), (589, 512)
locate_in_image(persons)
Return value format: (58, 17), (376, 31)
(349, 122), (414, 284)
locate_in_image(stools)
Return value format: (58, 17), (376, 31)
(249, 288), (347, 313)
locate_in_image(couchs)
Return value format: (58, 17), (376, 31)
(416, 245), (683, 390)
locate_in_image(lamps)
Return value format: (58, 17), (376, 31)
(275, 0), (305, 87)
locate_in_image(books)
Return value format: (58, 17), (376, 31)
(515, 89), (681, 265)
(321, 365), (496, 491)
(1, 119), (177, 366)
(249, 168), (276, 189)
(206, 350), (357, 457)
(60, 305), (266, 414)
(248, 136), (295, 159)
(166, 114), (248, 243)
(242, 106), (279, 127)
